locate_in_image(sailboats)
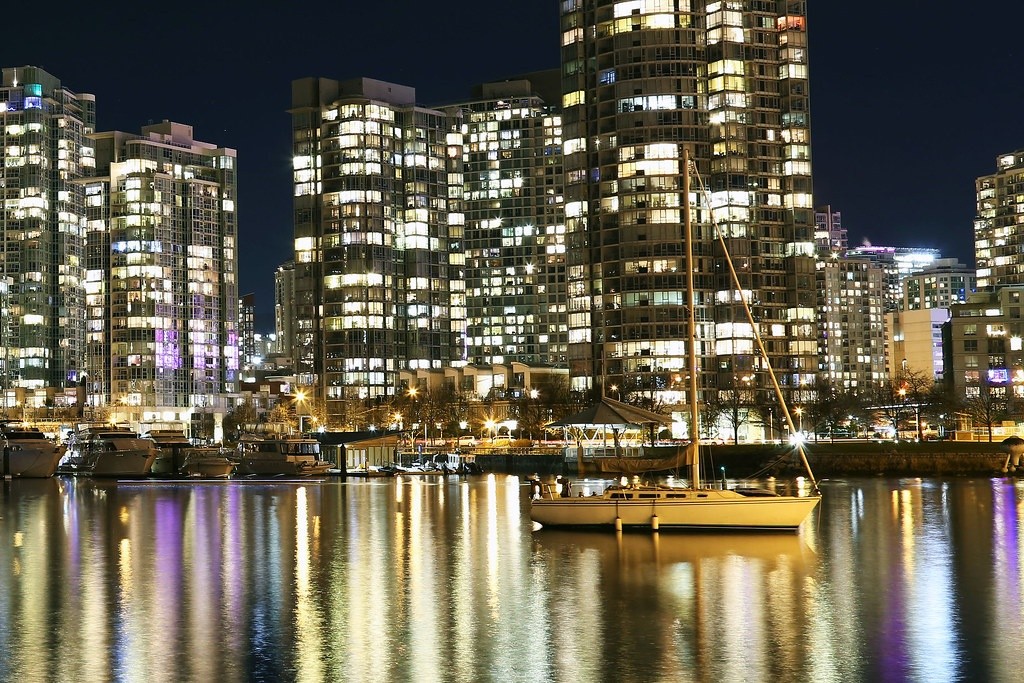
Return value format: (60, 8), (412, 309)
(1, 336), (337, 480)
(525, 148), (824, 534)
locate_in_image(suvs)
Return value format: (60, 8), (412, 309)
(454, 435), (476, 448)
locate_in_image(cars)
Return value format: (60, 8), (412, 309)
(699, 437), (727, 447)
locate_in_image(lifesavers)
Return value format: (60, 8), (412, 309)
(529, 482), (543, 498)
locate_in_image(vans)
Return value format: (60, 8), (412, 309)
(487, 435), (516, 446)
